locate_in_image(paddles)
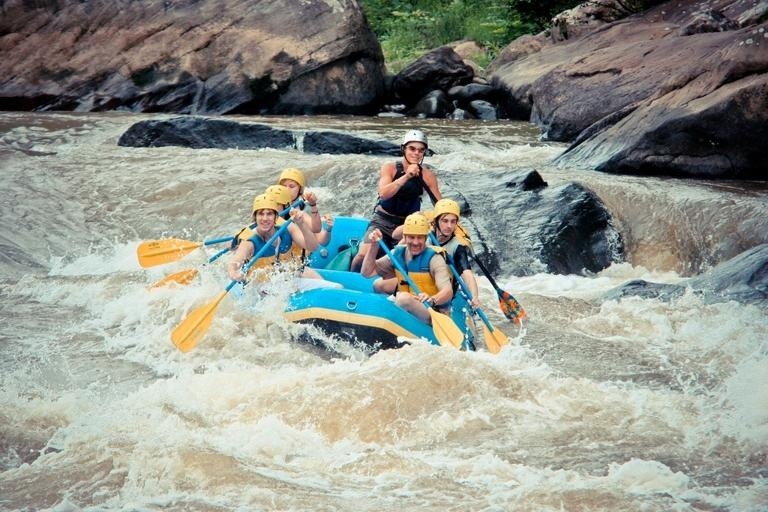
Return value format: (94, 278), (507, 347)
(426, 226), (509, 354)
(410, 164), (528, 327)
(146, 195), (305, 293)
(168, 207), (298, 354)
(373, 232), (464, 349)
(137, 235), (237, 268)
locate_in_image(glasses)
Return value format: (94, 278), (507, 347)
(404, 145), (425, 152)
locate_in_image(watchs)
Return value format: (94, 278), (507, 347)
(431, 298), (436, 307)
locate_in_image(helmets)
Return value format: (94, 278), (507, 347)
(404, 214), (429, 235)
(279, 168), (304, 188)
(253, 193), (279, 216)
(401, 131), (428, 151)
(434, 199), (460, 220)
(265, 186), (291, 205)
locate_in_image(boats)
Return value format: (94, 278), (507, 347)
(233, 216), (476, 359)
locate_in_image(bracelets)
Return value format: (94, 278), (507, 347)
(326, 228), (331, 232)
(309, 204), (316, 206)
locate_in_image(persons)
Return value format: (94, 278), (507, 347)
(227, 194), (321, 282)
(361, 214), (453, 324)
(278, 168), (322, 234)
(350, 130), (443, 272)
(392, 199), (479, 313)
(237, 185), (334, 261)
